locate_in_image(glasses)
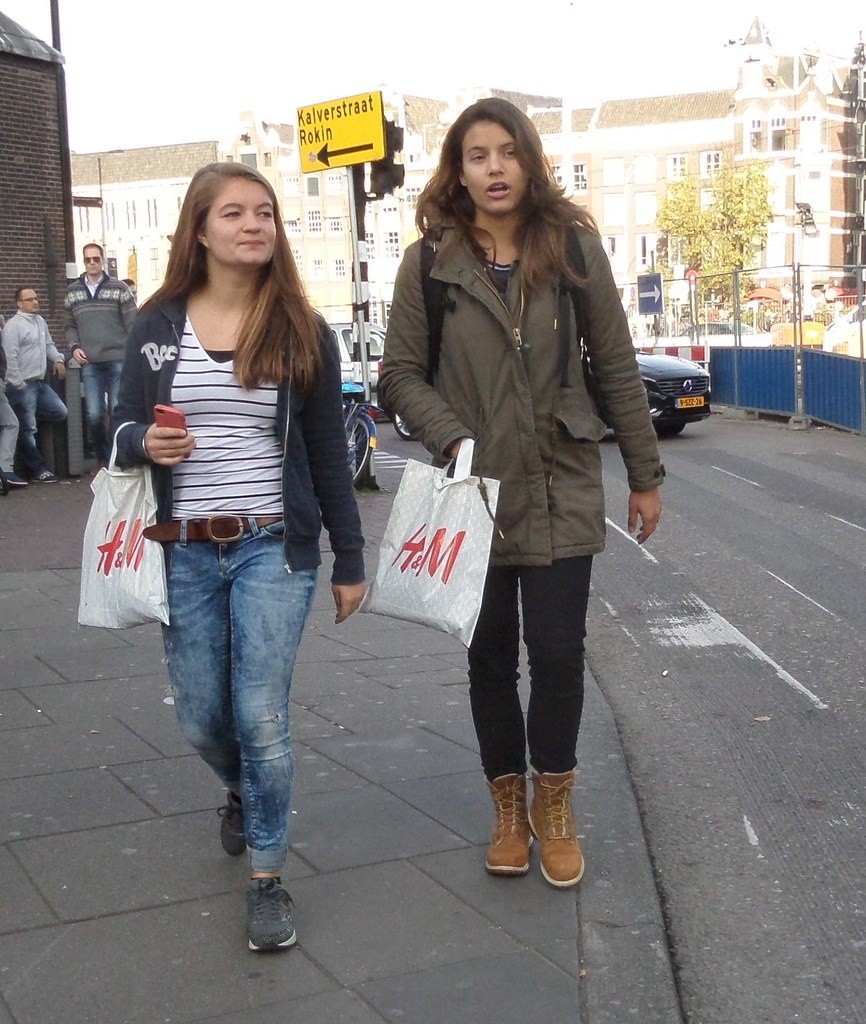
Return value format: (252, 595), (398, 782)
(20, 297), (39, 302)
(85, 256), (103, 263)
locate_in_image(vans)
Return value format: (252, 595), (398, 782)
(329, 321), (387, 407)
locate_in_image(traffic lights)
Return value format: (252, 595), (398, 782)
(370, 115), (404, 193)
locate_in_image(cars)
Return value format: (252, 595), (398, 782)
(643, 321), (772, 347)
(376, 346), (711, 442)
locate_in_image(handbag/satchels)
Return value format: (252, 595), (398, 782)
(75, 421), (170, 629)
(358, 434), (499, 647)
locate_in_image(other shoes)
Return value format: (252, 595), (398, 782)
(3, 469), (29, 486)
(29, 466), (58, 482)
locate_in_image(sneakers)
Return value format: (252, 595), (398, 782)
(245, 874), (297, 952)
(217, 783), (249, 855)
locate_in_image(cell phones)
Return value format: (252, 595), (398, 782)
(153, 405), (190, 458)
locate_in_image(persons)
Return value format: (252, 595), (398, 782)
(1, 286), (68, 483)
(377, 97), (667, 886)
(105, 162), (366, 951)
(123, 278), (137, 304)
(0, 345), (30, 484)
(62, 243), (138, 474)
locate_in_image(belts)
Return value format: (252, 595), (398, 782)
(142, 509), (281, 545)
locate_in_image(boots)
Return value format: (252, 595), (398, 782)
(484, 771), (537, 875)
(528, 762), (585, 887)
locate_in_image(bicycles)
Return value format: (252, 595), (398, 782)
(340, 383), (386, 487)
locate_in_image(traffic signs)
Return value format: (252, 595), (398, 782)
(296, 90), (388, 174)
